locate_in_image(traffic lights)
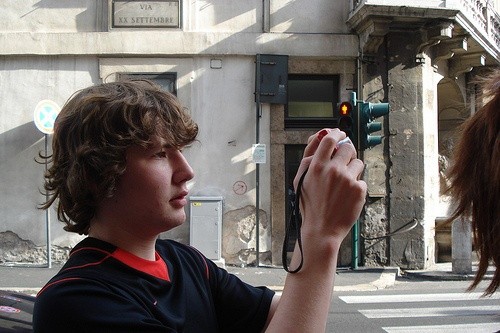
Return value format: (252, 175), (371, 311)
(334, 100), (390, 269)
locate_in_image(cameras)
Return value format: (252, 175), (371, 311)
(318, 130), (358, 166)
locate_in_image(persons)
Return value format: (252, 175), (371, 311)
(33, 72), (368, 333)
(431, 67), (500, 333)
